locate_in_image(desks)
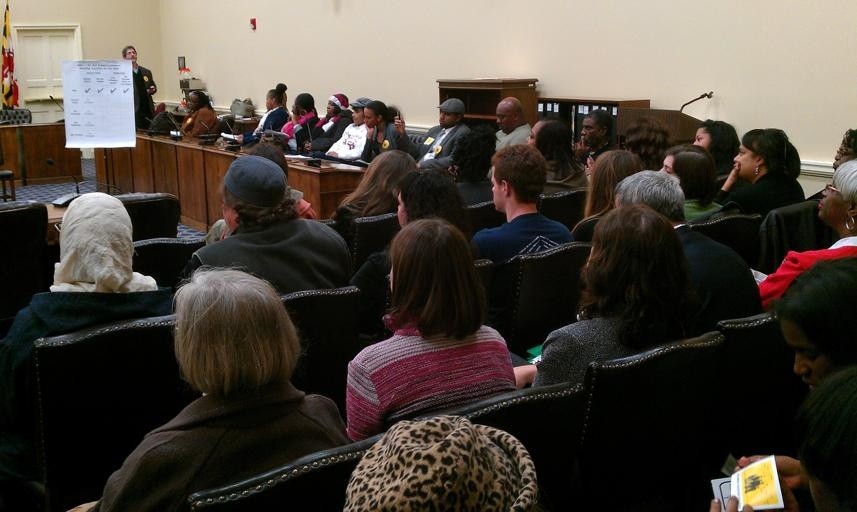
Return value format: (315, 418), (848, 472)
(18, 118), (87, 186)
(0, 115), (25, 191)
(91, 121), (376, 239)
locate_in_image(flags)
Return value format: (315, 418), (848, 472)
(0, 0), (20, 111)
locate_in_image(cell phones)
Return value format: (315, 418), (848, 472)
(398, 111), (400, 120)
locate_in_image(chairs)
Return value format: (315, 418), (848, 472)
(402, 131), (427, 152)
(149, 109), (290, 153)
(0, 109), (32, 127)
(0, 180), (855, 509)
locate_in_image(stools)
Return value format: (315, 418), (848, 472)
(0, 169), (18, 204)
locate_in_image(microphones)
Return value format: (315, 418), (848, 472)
(304, 117), (321, 167)
(224, 118), (241, 151)
(49, 95), (63, 111)
(668, 91), (713, 111)
(198, 120), (215, 146)
(44, 158), (81, 207)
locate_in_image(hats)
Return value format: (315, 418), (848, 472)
(223, 155), (289, 209)
(342, 413), (536, 512)
(437, 98), (466, 115)
(330, 94), (349, 111)
(350, 98), (373, 108)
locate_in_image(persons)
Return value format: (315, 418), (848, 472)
(234, 83), (406, 164)
(180, 91), (219, 139)
(340, 96), (856, 511)
(1, 145), (369, 512)
(121, 46), (157, 130)
